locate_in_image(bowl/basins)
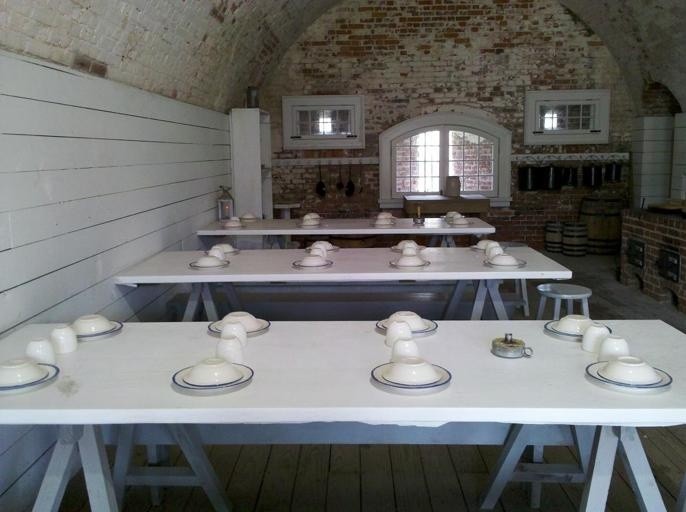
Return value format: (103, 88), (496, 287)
(557, 314), (593, 335)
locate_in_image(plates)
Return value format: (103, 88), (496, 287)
(386, 240), (431, 271)
(469, 239), (527, 271)
(189, 243), (240, 270)
(369, 212), (397, 228)
(170, 310), (270, 390)
(292, 240), (339, 269)
(439, 212), (474, 226)
(544, 319), (673, 390)
(295, 212), (323, 230)
(374, 310), (452, 388)
(221, 212), (258, 229)
(0, 314), (124, 392)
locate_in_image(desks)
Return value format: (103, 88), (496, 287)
(402, 194), (490, 215)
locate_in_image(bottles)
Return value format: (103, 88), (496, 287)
(247, 87), (259, 107)
(217, 184), (234, 221)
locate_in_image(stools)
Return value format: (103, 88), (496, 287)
(536, 281), (593, 321)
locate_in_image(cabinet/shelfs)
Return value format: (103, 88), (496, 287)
(229, 108), (273, 249)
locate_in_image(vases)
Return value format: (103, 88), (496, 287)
(445, 176), (460, 197)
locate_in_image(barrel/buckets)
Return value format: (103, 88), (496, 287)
(578, 195), (630, 256)
(550, 155), (579, 191)
(581, 155), (602, 188)
(518, 156), (551, 191)
(604, 155), (622, 183)
(563, 221), (589, 257)
(273, 202), (301, 209)
(545, 220), (567, 253)
